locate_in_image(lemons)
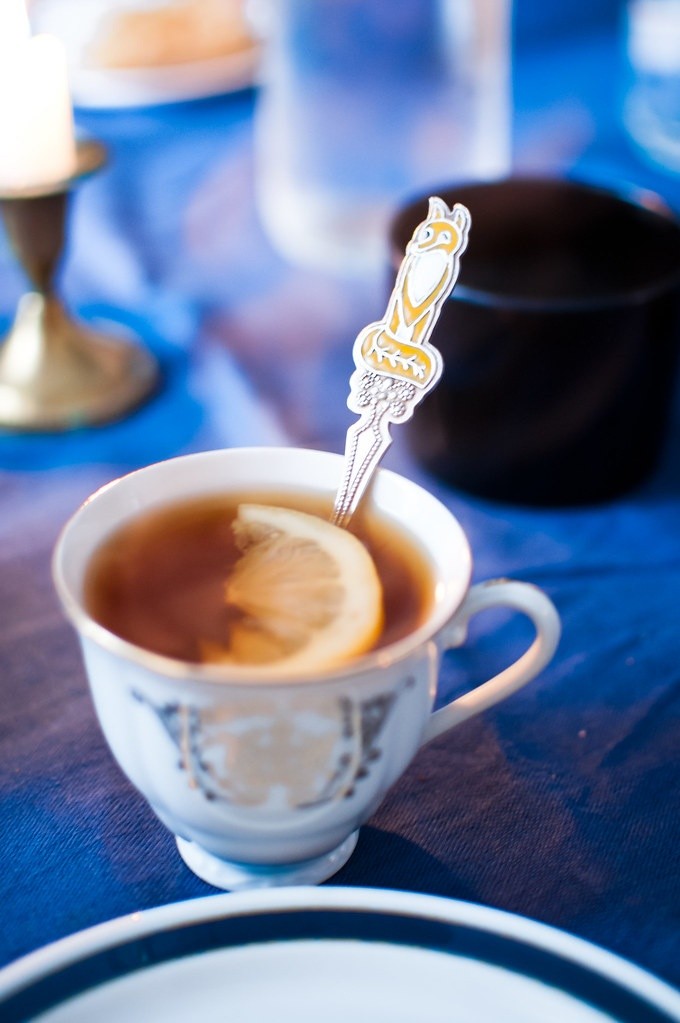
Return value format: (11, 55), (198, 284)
(200, 503), (381, 669)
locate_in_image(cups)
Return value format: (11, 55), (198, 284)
(251, 0), (506, 288)
(387, 176), (680, 510)
(51, 442), (560, 893)
(625, 0), (680, 181)
(33, 0), (269, 109)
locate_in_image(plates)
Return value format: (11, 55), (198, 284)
(0, 884), (680, 1023)
